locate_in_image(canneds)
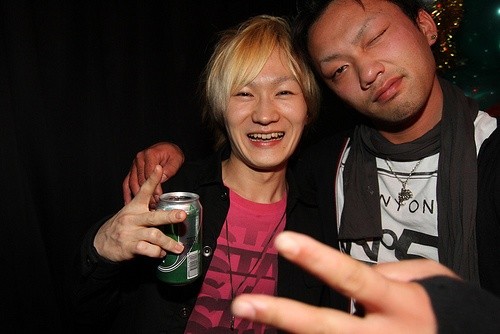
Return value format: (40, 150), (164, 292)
(153, 192), (202, 286)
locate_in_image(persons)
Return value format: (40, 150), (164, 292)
(68, 14), (349, 333)
(122, 0), (500, 334)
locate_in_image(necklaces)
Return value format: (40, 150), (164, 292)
(216, 210), (290, 331)
(384, 157), (431, 202)
